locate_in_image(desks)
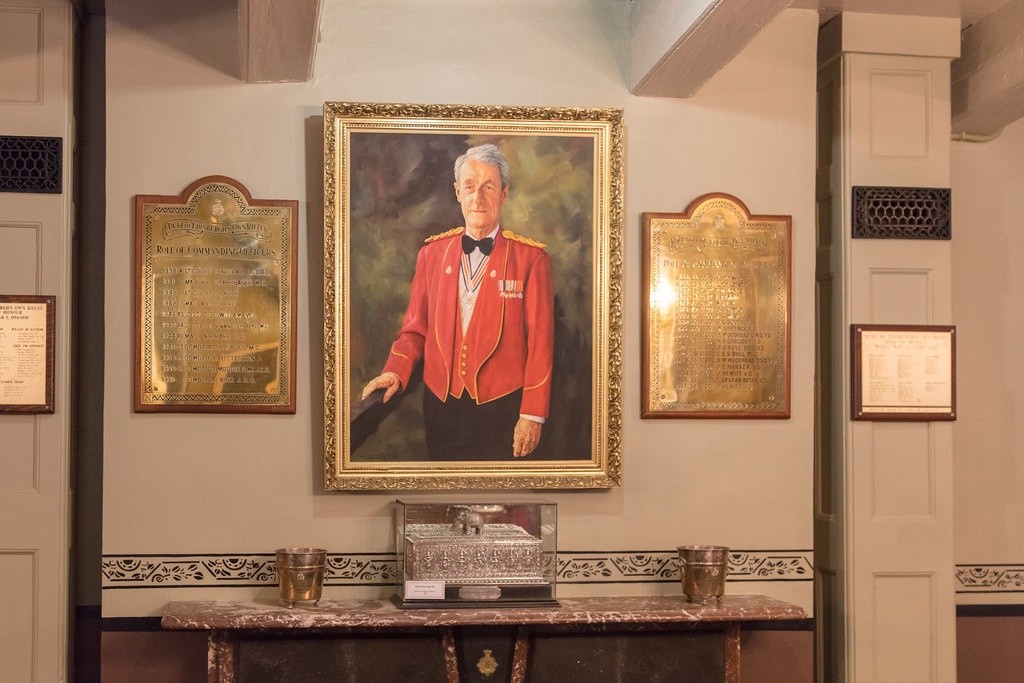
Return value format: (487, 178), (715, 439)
(157, 591), (809, 683)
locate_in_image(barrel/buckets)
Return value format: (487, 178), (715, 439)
(675, 544), (730, 597)
(272, 548), (325, 606)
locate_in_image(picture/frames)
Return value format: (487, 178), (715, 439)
(320, 96), (630, 494)
